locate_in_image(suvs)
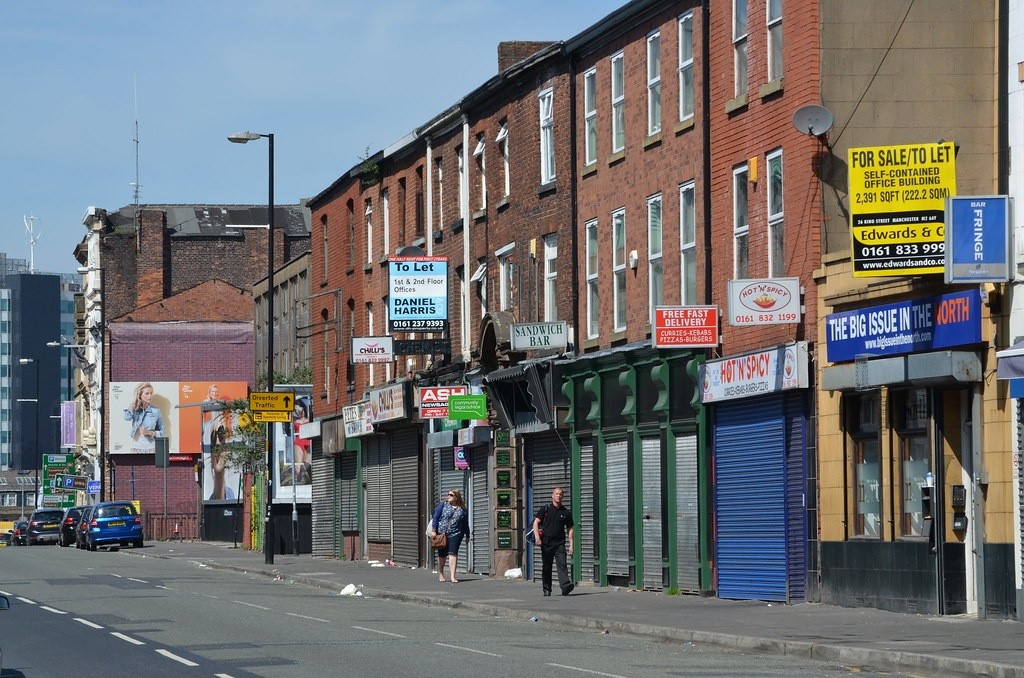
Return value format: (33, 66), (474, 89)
(24, 507), (65, 546)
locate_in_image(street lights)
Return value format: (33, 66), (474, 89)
(227, 132), (277, 566)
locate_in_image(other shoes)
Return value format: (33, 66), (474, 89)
(563, 584), (575, 595)
(544, 591), (551, 597)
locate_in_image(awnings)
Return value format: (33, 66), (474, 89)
(994, 340), (1024, 379)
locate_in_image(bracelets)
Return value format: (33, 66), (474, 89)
(151, 431), (155, 438)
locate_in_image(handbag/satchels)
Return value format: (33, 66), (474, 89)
(525, 504), (548, 545)
(425, 518), (434, 536)
(430, 532), (446, 548)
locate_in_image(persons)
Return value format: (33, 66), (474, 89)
(533, 488), (575, 596)
(293, 399), (311, 474)
(431, 489), (470, 583)
(208, 453), (235, 500)
(203, 384), (228, 452)
(123, 383), (165, 453)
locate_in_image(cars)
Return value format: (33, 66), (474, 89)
(82, 499), (143, 552)
(8, 521), (30, 546)
(74, 506), (91, 550)
(57, 506), (88, 547)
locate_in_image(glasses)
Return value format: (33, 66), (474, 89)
(448, 494), (456, 497)
